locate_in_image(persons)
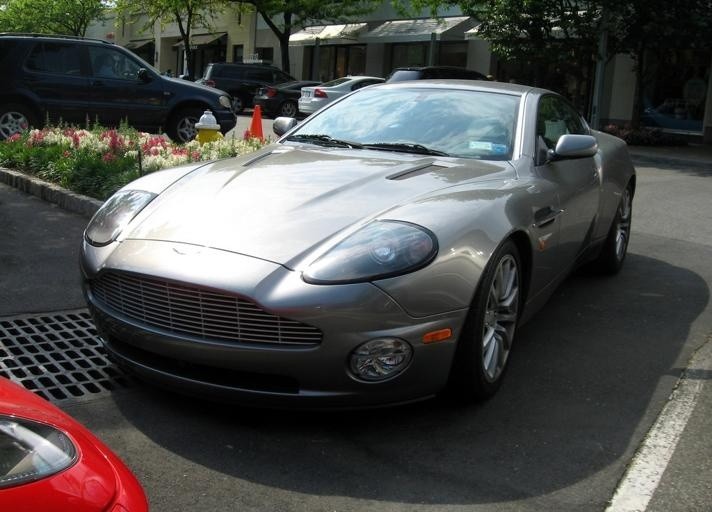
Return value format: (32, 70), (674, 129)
(100, 54), (115, 76)
